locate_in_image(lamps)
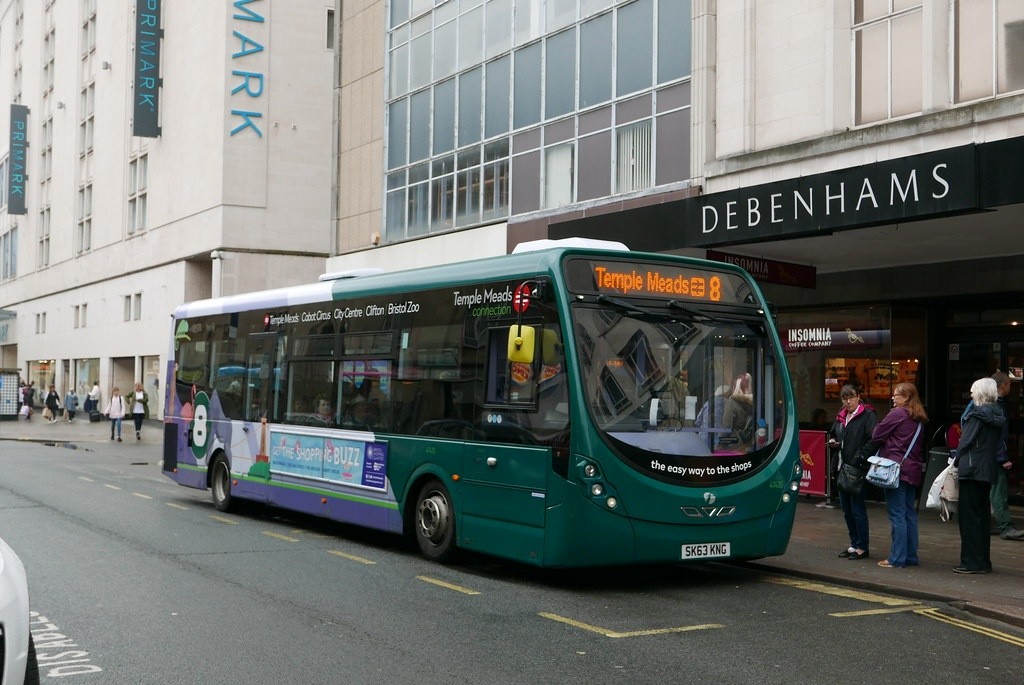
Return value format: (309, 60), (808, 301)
(102, 61), (111, 70)
(58, 102), (65, 109)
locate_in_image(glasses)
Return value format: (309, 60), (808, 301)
(893, 394), (902, 397)
(842, 395), (857, 401)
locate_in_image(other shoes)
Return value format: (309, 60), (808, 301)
(136, 432), (140, 439)
(989, 527), (1024, 540)
(53, 420), (58, 424)
(952, 560), (992, 574)
(68, 420), (71, 423)
(48, 419), (52, 424)
(111, 436), (114, 440)
(837, 546), (869, 560)
(117, 438), (121, 441)
(877, 559), (903, 569)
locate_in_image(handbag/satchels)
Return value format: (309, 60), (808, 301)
(833, 463), (871, 498)
(42, 407), (52, 419)
(865, 455), (901, 489)
(19, 405), (30, 416)
(939, 458), (960, 503)
(925, 456), (959, 523)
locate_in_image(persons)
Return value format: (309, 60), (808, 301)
(952, 373), (1024, 574)
(872, 383), (930, 568)
(64, 387), (78, 422)
(88, 381), (100, 410)
(103, 387), (126, 441)
(19, 379), (38, 419)
(641, 352), (757, 452)
(344, 395), (383, 427)
(309, 393), (335, 426)
(827, 385), (886, 560)
(125, 382), (149, 440)
(44, 385), (61, 424)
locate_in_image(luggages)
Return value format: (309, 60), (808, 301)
(90, 409), (101, 423)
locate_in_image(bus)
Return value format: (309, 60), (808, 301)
(159, 236), (799, 566)
